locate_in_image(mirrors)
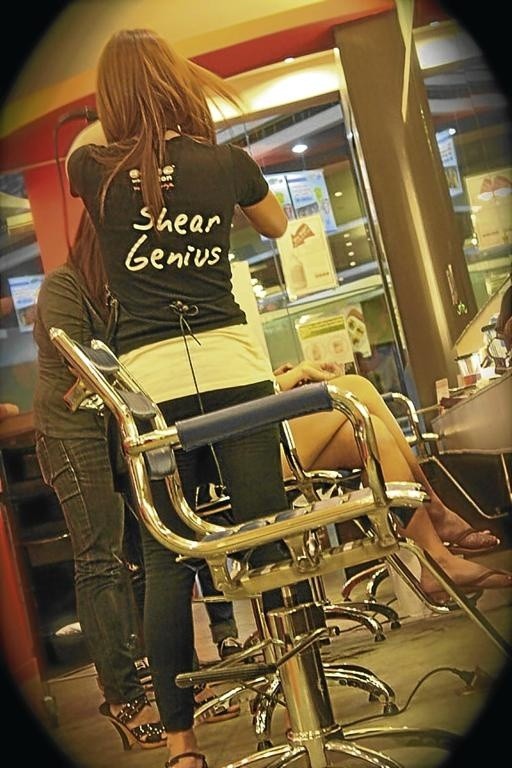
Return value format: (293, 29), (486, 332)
(401, 2), (511, 342)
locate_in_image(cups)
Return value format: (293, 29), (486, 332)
(455, 352), (482, 386)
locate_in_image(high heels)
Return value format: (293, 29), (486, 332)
(98, 694), (167, 751)
(192, 683), (241, 722)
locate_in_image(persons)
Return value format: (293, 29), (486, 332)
(272, 355), (510, 600)
(29, 204), (242, 752)
(66, 26), (292, 767)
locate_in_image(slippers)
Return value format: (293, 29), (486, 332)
(446, 530), (503, 555)
(429, 568), (511, 600)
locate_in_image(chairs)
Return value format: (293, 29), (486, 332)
(51, 330), (508, 765)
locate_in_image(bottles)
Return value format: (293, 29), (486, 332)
(288, 251), (306, 289)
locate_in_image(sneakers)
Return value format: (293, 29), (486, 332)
(218, 636), (244, 660)
